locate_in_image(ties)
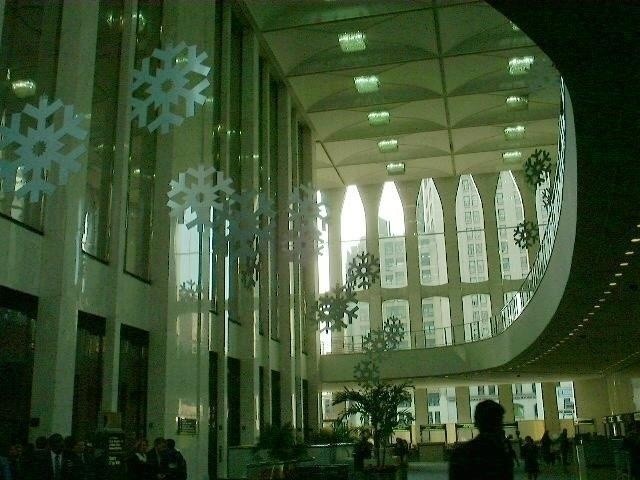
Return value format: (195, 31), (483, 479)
(54, 455), (60, 480)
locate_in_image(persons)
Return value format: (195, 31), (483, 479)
(558, 427), (572, 463)
(520, 435), (540, 480)
(448, 399), (515, 480)
(621, 419), (640, 480)
(539, 429), (553, 463)
(0, 430), (188, 480)
